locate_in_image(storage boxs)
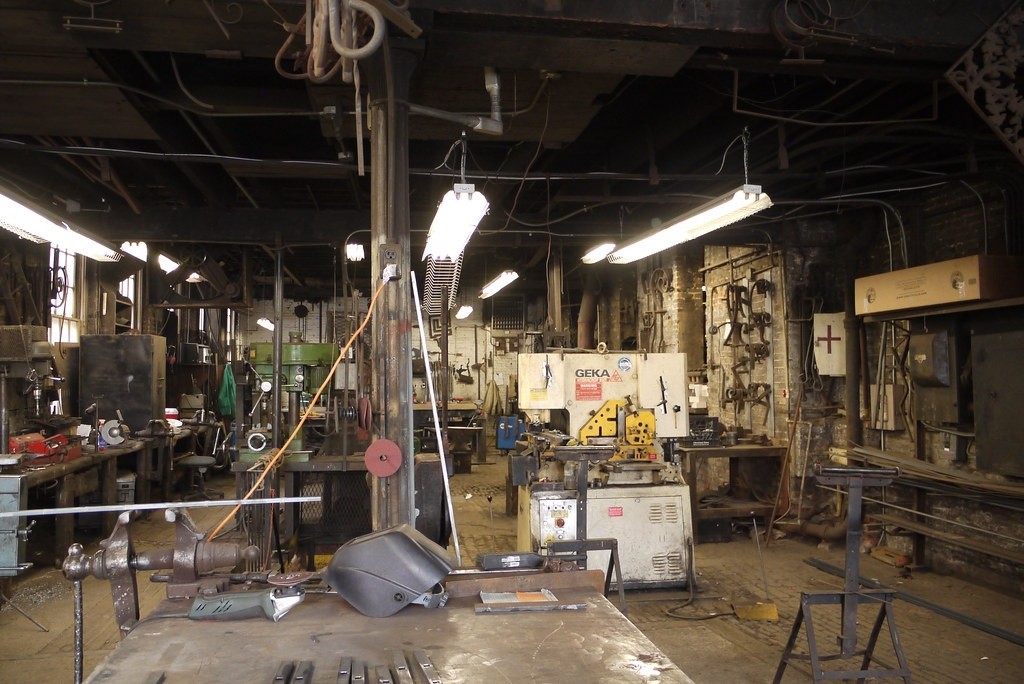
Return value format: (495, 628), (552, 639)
(854, 255), (1024, 317)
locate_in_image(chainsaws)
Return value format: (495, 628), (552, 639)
(186, 571), (314, 623)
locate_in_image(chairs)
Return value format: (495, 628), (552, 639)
(178, 432), (234, 502)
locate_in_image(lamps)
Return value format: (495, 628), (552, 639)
(606, 131), (775, 264)
(421, 139), (489, 263)
(0, 185), (124, 262)
(478, 269), (520, 300)
